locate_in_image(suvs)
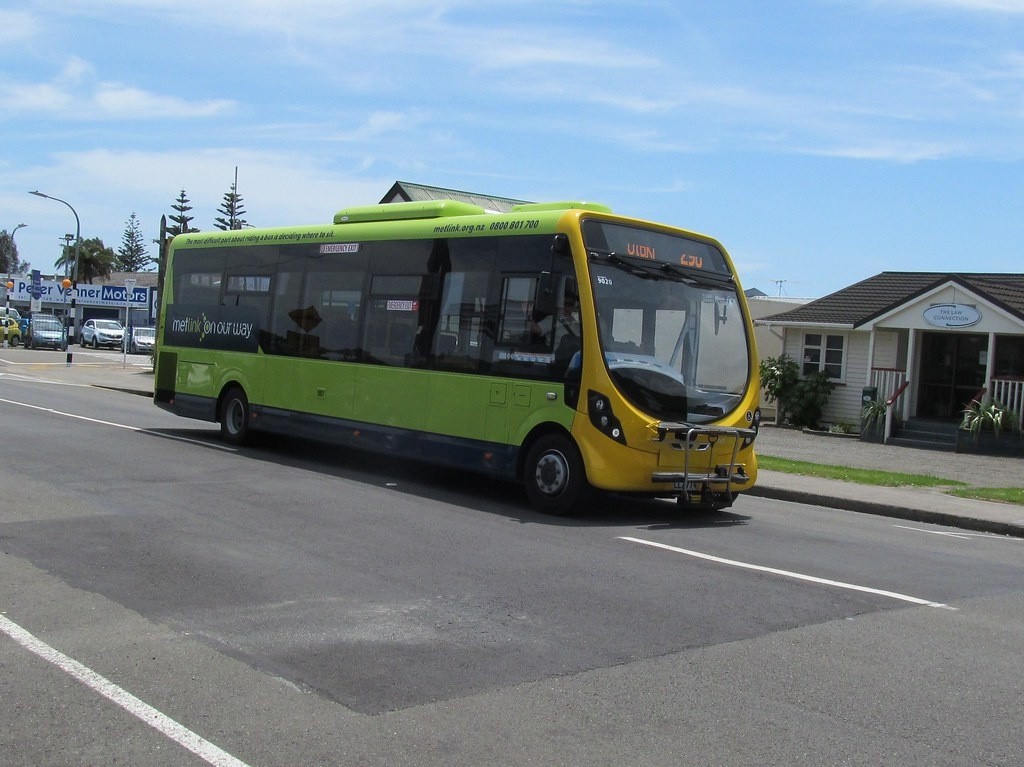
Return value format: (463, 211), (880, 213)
(80, 318), (126, 349)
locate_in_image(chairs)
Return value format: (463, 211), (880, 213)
(184, 284), (457, 368)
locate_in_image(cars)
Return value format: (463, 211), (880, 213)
(120, 326), (156, 354)
(0, 305), (68, 351)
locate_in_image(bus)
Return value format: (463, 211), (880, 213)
(152, 198), (760, 515)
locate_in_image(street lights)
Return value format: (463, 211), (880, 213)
(28, 190), (81, 368)
(4, 224), (28, 348)
(57, 232), (76, 350)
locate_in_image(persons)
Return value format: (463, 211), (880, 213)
(521, 290), (580, 354)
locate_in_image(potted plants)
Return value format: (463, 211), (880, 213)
(957, 396), (1021, 456)
(860, 391), (901, 443)
